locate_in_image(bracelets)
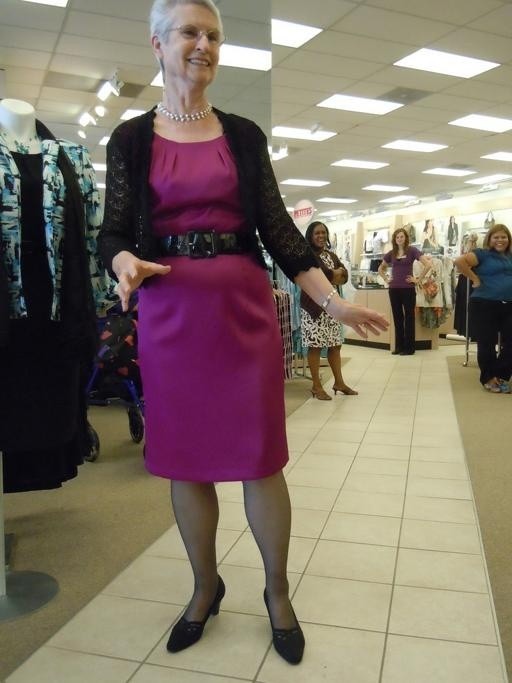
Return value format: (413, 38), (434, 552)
(321, 289), (336, 312)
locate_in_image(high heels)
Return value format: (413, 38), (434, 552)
(333, 383), (358, 395)
(264, 588), (305, 664)
(166, 574), (225, 653)
(310, 389), (332, 400)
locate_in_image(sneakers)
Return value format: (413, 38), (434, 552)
(484, 381), (500, 393)
(495, 378), (511, 393)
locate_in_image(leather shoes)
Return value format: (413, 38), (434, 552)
(392, 351), (399, 354)
(400, 351), (407, 355)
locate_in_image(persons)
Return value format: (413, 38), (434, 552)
(422, 219), (437, 242)
(97, 0), (390, 665)
(0, 97), (95, 493)
(378, 228), (431, 355)
(454, 224), (512, 394)
(300, 221), (358, 400)
(447, 216), (458, 246)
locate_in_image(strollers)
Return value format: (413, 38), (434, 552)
(86, 292), (144, 464)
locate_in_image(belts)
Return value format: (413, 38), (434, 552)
(158, 228), (248, 260)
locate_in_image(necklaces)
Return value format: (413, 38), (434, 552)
(157, 101), (212, 122)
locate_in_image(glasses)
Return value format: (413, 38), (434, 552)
(166, 24), (221, 46)
